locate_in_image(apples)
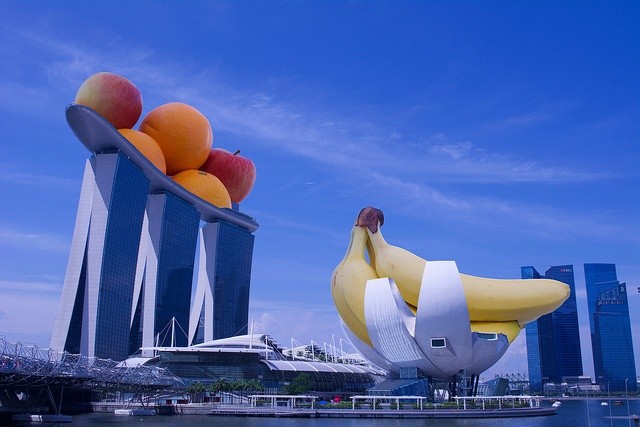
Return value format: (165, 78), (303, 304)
(198, 147), (257, 203)
(75, 71), (142, 129)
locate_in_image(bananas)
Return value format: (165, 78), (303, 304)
(331, 207), (571, 351)
